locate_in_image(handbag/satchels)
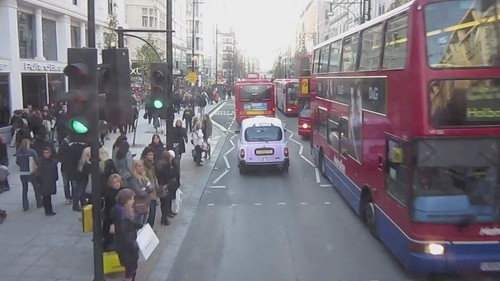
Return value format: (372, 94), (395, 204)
(156, 184), (169, 199)
(135, 202), (149, 217)
(28, 156), (38, 173)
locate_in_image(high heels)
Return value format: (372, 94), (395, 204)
(161, 217), (171, 226)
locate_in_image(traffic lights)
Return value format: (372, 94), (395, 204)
(152, 69), (166, 111)
(98, 65), (114, 122)
(64, 62), (95, 135)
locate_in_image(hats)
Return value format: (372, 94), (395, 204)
(168, 149), (175, 158)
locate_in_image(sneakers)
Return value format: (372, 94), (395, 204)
(65, 199), (71, 205)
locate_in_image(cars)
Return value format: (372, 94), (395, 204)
(236, 115), (290, 174)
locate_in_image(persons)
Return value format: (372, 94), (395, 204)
(111, 188), (142, 281)
(146, 130), (161, 155)
(105, 172), (125, 252)
(167, 150), (182, 218)
(190, 115), (205, 162)
(16, 139), (44, 211)
(115, 140), (133, 180)
(155, 148), (175, 226)
(72, 144), (99, 211)
(141, 148), (159, 235)
(109, 80), (153, 133)
(181, 106), (194, 135)
(151, 111), (160, 132)
(59, 133), (76, 205)
(65, 141), (84, 213)
(0, 134), (10, 220)
(173, 118), (188, 157)
(112, 136), (127, 170)
(172, 76), (234, 111)
(190, 122), (204, 166)
(94, 141), (117, 242)
(99, 120), (109, 146)
(126, 158), (153, 231)
(36, 147), (59, 215)
(8, 100), (67, 146)
(200, 113), (211, 160)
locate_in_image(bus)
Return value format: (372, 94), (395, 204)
(235, 79), (276, 125)
(312, 0), (499, 280)
(298, 54), (312, 137)
(245, 72), (259, 78)
(274, 78), (299, 114)
